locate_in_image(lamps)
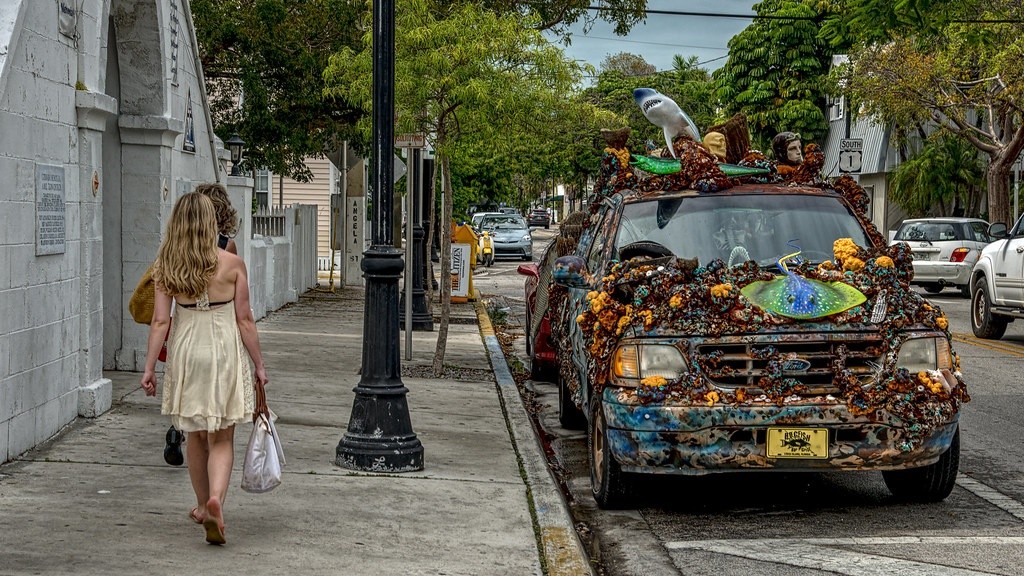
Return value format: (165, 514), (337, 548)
(226, 132), (247, 176)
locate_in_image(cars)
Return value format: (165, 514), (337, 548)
(470, 213), (537, 262)
(548, 187), (962, 511)
(889, 217), (1003, 299)
(516, 234), (560, 382)
(467, 207), (528, 233)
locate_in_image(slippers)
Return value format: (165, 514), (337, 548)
(203, 518), (226, 544)
(190, 507), (203, 524)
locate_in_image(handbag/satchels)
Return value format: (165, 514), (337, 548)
(240, 379), (286, 493)
(129, 258), (158, 325)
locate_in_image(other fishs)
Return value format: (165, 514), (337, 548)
(628, 155), (771, 178)
(740, 236), (869, 319)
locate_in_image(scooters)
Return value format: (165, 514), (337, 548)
(471, 225), (500, 267)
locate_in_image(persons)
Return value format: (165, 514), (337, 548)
(157, 182), (238, 466)
(140, 192), (267, 547)
(773, 131), (802, 165)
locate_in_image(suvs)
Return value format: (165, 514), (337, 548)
(969, 210), (1024, 341)
(525, 209), (551, 229)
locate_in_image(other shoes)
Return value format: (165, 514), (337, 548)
(164, 425), (185, 466)
